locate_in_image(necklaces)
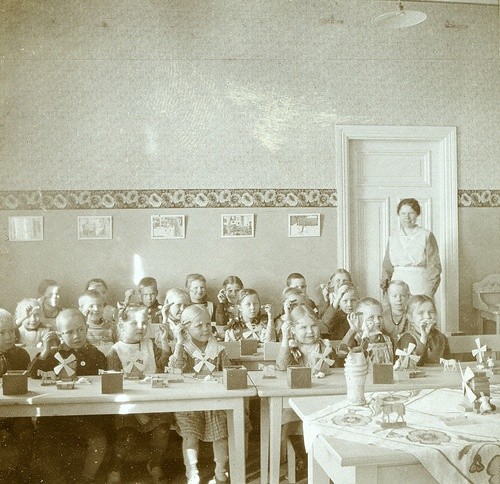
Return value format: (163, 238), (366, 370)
(391, 310), (404, 330)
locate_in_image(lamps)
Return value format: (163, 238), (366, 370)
(375, 0), (427, 28)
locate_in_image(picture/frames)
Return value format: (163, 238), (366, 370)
(77, 216), (112, 239)
(151, 215), (186, 239)
(221, 213), (255, 238)
(9, 216), (44, 241)
(288, 213), (321, 238)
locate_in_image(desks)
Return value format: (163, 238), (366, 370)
(0, 370), (257, 484)
(21, 342), (240, 458)
(232, 330), (500, 463)
(288, 383), (500, 484)
(247, 357), (500, 484)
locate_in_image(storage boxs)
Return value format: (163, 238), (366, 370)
(238, 338), (257, 355)
(372, 363), (393, 383)
(102, 371), (124, 394)
(287, 366), (311, 389)
(2, 370), (30, 395)
(222, 365), (248, 390)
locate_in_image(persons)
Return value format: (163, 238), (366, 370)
(0, 262), (451, 484)
(380, 199), (442, 300)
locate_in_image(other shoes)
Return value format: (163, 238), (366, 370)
(18, 462), (34, 484)
(152, 467), (167, 484)
(285, 456), (307, 480)
(214, 469), (228, 484)
(107, 472), (120, 484)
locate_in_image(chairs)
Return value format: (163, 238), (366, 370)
(472, 274), (500, 335)
